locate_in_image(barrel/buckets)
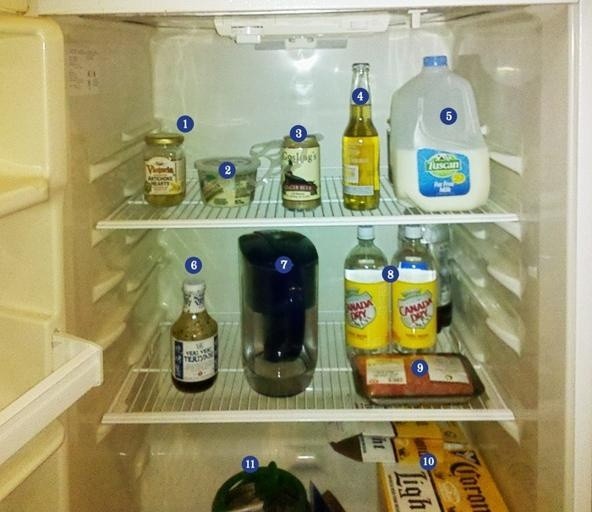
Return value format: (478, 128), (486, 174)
(388, 55), (491, 213)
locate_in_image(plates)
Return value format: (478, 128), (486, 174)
(210, 466), (306, 512)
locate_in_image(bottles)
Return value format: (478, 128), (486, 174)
(388, 224), (454, 357)
(341, 61), (381, 211)
(343, 224), (390, 361)
(169, 278), (221, 394)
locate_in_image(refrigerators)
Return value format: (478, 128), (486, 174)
(0, 0), (592, 512)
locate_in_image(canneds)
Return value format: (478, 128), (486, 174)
(279, 137), (322, 211)
(143, 131), (186, 208)
